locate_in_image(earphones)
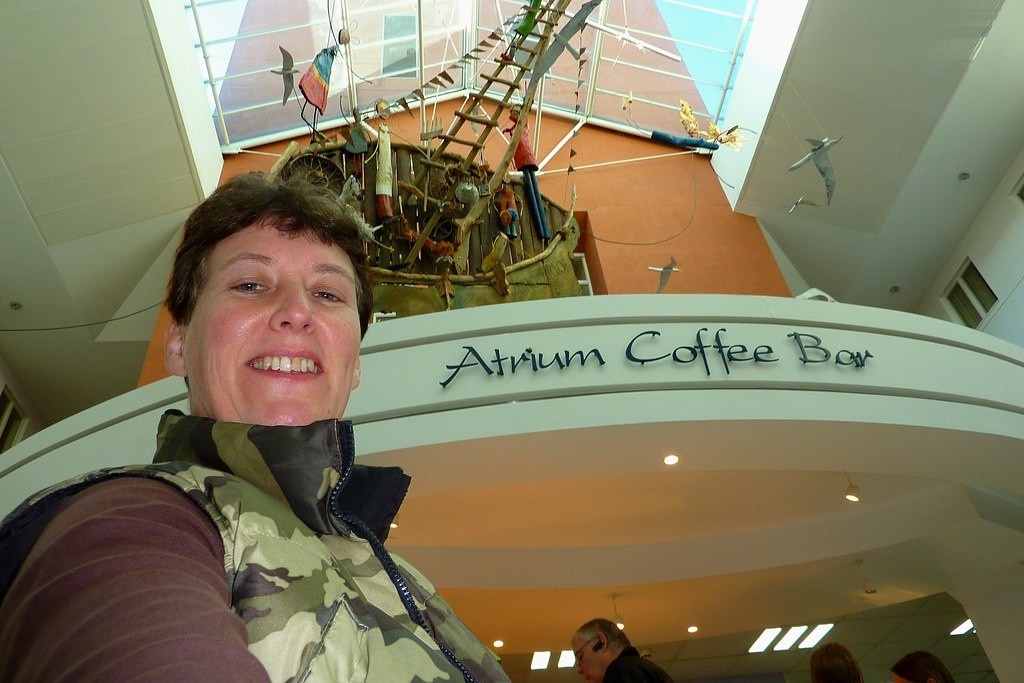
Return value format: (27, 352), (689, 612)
(592, 640), (605, 652)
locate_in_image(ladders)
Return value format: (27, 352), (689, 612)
(395, 0), (575, 274)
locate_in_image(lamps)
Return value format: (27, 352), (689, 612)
(610, 594), (626, 631)
(842, 472), (861, 504)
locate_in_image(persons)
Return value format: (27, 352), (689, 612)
(340, 94), (376, 176)
(296, 0), (372, 145)
(492, 170), (523, 239)
(890, 651), (956, 683)
(365, 100), (430, 229)
(809, 643), (864, 683)
(0, 174), (514, 683)
(503, 104), (551, 251)
(572, 618), (675, 683)
